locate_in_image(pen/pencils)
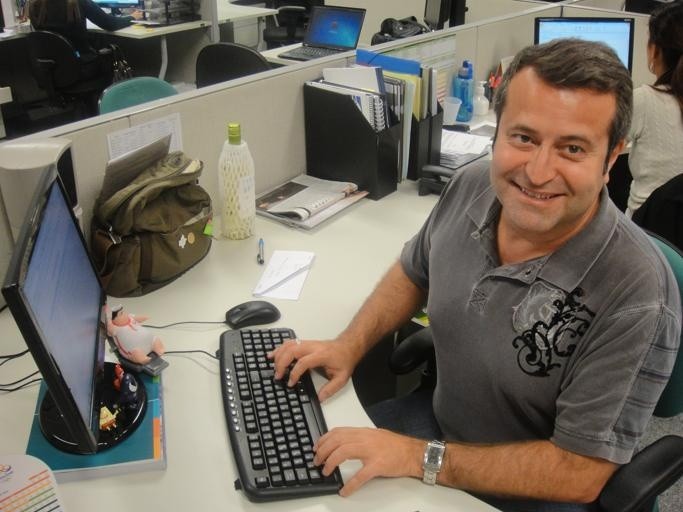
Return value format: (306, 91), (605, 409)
(16, 0), (26, 20)
(257, 238), (264, 264)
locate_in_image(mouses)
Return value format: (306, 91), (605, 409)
(226, 301), (280, 330)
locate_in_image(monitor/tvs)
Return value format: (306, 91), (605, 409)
(534, 17), (634, 79)
(91, 0), (144, 17)
(423, 0), (450, 31)
(0, 164), (147, 454)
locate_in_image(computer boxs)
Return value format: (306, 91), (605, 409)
(0, 139), (83, 297)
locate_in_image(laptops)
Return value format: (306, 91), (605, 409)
(278, 3), (366, 62)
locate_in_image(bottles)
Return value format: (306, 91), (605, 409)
(452, 60), (475, 121)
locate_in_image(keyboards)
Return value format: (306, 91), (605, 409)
(220, 328), (344, 503)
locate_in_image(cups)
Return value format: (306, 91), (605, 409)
(441, 96), (462, 123)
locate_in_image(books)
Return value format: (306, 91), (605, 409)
(256, 49), (437, 229)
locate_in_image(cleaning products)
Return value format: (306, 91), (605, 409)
(472, 80), (490, 116)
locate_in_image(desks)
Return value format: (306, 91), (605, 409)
(0, 2), (682, 512)
(86, 18), (208, 79)
(256, 38), (306, 66)
(216, 1), (279, 49)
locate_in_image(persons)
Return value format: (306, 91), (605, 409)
(28, 1), (146, 90)
(623, 0), (683, 218)
(103, 304), (168, 365)
(267, 40), (683, 512)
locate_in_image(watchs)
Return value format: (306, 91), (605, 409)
(422, 439), (447, 485)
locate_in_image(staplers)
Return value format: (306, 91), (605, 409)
(443, 124), (471, 135)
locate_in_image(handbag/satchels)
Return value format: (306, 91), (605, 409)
(97, 150), (212, 296)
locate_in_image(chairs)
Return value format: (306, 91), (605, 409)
(621, 172), (682, 252)
(393, 229), (682, 512)
(0, 30), (121, 125)
(262, 2), (313, 46)
(93, 78), (177, 113)
(195, 43), (269, 88)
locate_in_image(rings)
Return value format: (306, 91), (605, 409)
(296, 338), (302, 347)
